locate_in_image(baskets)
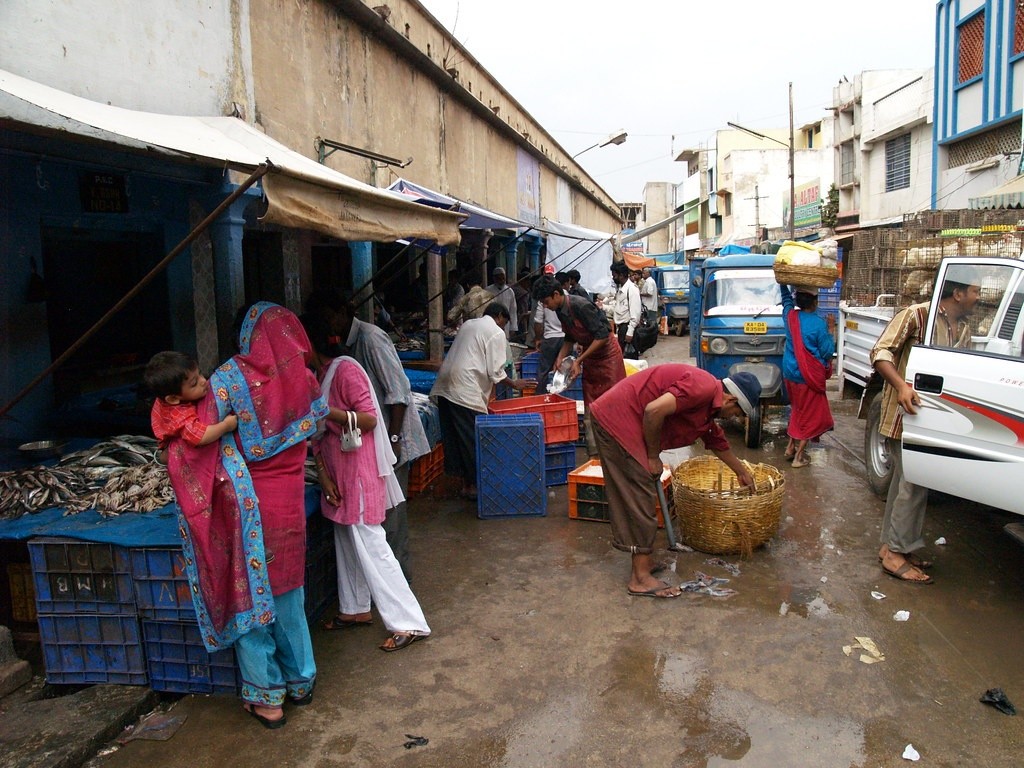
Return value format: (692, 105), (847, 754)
(772, 245), (839, 289)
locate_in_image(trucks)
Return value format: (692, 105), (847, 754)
(839, 249), (1024, 540)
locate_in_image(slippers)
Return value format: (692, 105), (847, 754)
(292, 692), (313, 706)
(878, 553), (932, 568)
(650, 561), (667, 575)
(381, 633), (424, 651)
(627, 581), (681, 597)
(882, 560), (933, 585)
(244, 703), (286, 729)
(324, 614), (374, 630)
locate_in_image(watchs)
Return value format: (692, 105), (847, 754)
(389, 435), (403, 443)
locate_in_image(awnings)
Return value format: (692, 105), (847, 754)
(0, 69), (468, 420)
(719, 244), (751, 256)
(750, 233), (820, 255)
(614, 251), (657, 272)
(368, 178), (603, 331)
(542, 220), (620, 298)
(968, 175), (1024, 209)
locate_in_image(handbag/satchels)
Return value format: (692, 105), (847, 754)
(633, 320), (658, 354)
(341, 411), (363, 452)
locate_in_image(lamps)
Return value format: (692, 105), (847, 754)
(572, 128), (627, 159)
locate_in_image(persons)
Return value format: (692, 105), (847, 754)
(429, 302), (538, 499)
(303, 314), (432, 652)
(144, 351), (236, 449)
(589, 364), (763, 598)
(611, 261), (643, 360)
(159, 302), (331, 729)
(309, 298), (432, 593)
(780, 285), (835, 469)
(373, 263), (594, 395)
(871, 264), (980, 584)
(531, 277), (628, 468)
(627, 269), (659, 321)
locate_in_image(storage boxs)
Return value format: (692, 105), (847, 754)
(27, 349), (672, 699)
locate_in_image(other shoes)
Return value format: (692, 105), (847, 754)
(784, 450), (795, 460)
(792, 458), (808, 467)
(459, 486), (477, 500)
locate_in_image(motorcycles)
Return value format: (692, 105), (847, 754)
(686, 252), (811, 448)
(649, 265), (690, 337)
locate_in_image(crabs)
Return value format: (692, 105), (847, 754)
(96, 458), (177, 513)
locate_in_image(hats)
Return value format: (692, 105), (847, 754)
(493, 267), (505, 275)
(724, 372), (762, 415)
(544, 264), (555, 274)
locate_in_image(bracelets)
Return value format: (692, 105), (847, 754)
(316, 462), (325, 476)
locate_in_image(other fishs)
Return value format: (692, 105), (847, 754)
(0, 434), (175, 520)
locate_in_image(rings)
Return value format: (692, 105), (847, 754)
(326, 496), (330, 500)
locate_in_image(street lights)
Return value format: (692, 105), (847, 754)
(726, 120), (795, 245)
